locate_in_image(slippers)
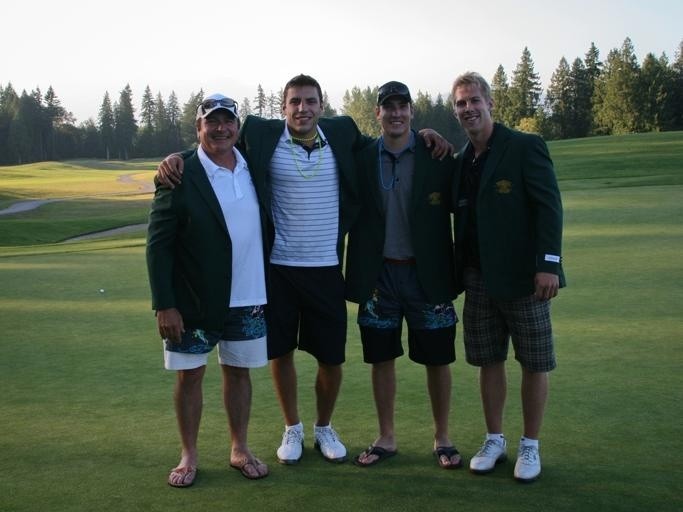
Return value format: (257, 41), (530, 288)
(353, 443), (398, 466)
(229, 453), (267, 481)
(167, 460), (198, 488)
(433, 440), (462, 468)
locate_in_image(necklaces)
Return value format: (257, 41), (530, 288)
(288, 132), (323, 181)
(376, 132), (417, 192)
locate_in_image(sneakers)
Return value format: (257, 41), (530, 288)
(469, 439), (541, 482)
(277, 422), (348, 464)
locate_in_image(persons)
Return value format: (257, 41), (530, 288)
(343, 80), (464, 471)
(447, 71), (569, 484)
(143, 94), (271, 488)
(155, 72), (455, 466)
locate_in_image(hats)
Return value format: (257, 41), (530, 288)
(195, 94), (238, 119)
(376, 81), (410, 105)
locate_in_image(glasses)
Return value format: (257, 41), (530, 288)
(201, 98), (237, 108)
